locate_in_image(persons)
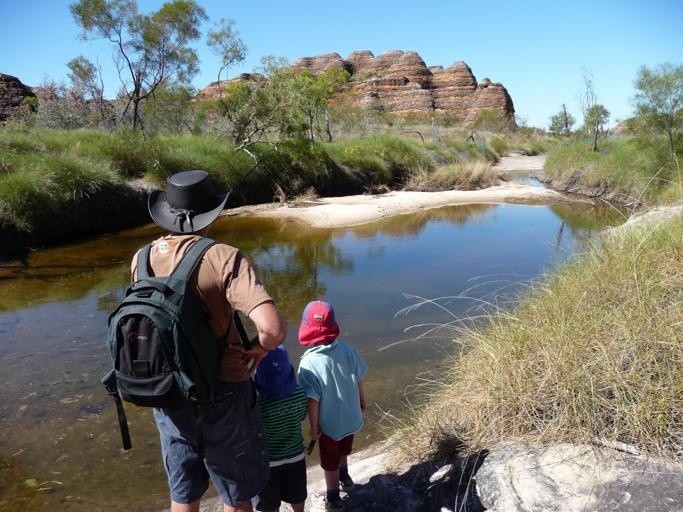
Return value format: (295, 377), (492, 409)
(296, 298), (367, 512)
(248, 334), (312, 512)
(125, 167), (289, 512)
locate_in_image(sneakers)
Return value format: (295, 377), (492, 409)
(339, 478), (354, 492)
(325, 496), (348, 511)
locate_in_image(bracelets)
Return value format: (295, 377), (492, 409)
(257, 342), (274, 354)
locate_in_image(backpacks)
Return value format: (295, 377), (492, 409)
(100, 236), (232, 452)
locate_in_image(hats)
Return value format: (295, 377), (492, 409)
(297, 300), (340, 347)
(146, 169), (234, 235)
(253, 343), (296, 401)
